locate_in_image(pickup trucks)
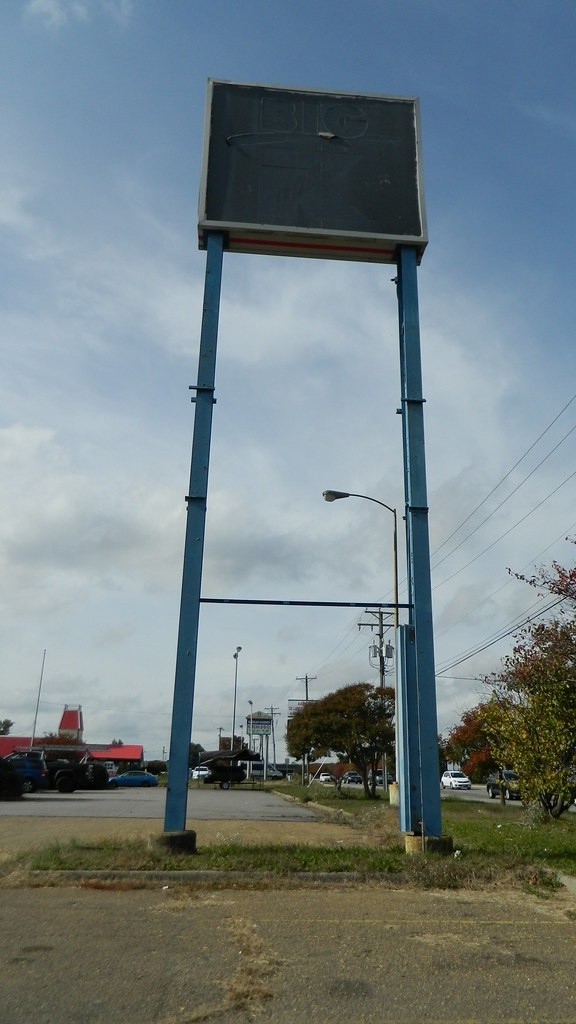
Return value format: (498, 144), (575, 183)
(26, 751), (96, 794)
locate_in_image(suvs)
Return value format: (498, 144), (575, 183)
(368, 768), (393, 787)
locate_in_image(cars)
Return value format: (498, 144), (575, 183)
(343, 771), (362, 785)
(305, 773), (313, 781)
(3, 752), (49, 794)
(441, 770), (472, 789)
(109, 770), (158, 788)
(0, 757), (25, 799)
(192, 766), (212, 780)
(320, 773), (332, 782)
(486, 769), (524, 800)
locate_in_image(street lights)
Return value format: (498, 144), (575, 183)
(322, 490), (399, 788)
(247, 700), (253, 783)
(231, 646), (242, 753)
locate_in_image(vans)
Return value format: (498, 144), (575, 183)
(237, 761), (285, 780)
